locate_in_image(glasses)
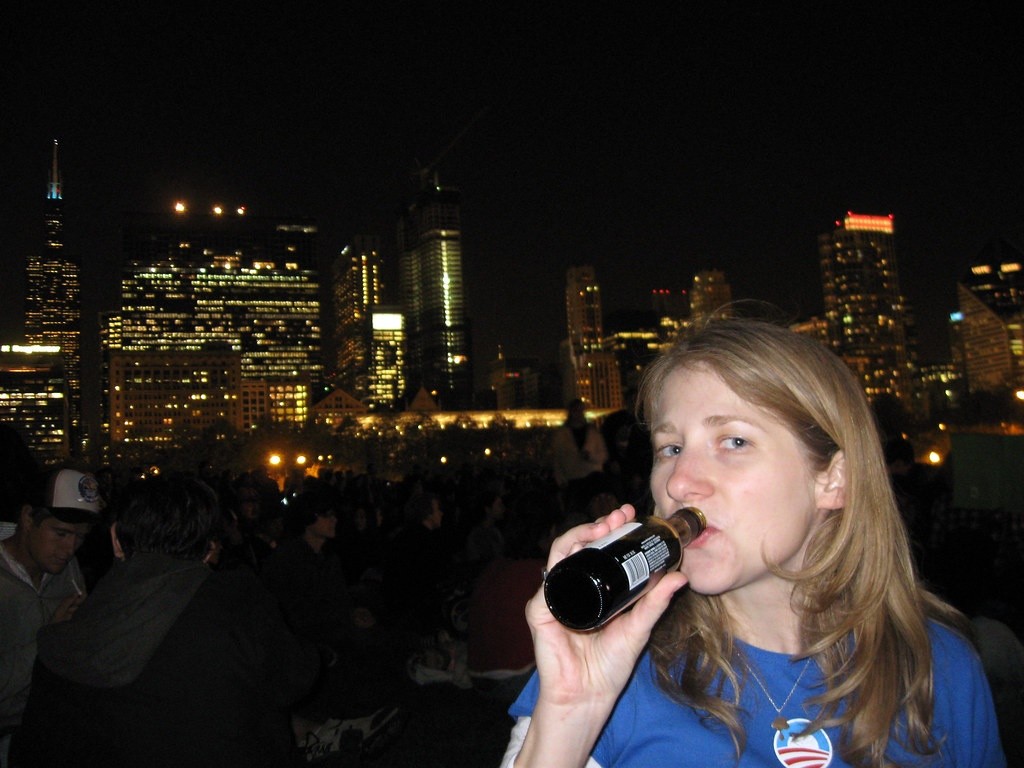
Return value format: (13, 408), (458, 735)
(315, 507), (339, 518)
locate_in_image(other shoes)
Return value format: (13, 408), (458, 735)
(474, 694), (516, 726)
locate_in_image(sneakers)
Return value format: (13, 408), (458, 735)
(295, 704), (399, 764)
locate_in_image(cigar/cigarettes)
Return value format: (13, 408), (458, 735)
(72, 580), (82, 596)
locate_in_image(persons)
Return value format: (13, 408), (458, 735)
(0, 386), (656, 768)
(883, 436), (1023, 768)
(496, 318), (1007, 767)
(548, 400), (608, 488)
(9, 470), (320, 767)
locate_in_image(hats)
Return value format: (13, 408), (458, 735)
(27, 463), (101, 523)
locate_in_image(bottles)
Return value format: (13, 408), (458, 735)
(544, 507), (706, 631)
